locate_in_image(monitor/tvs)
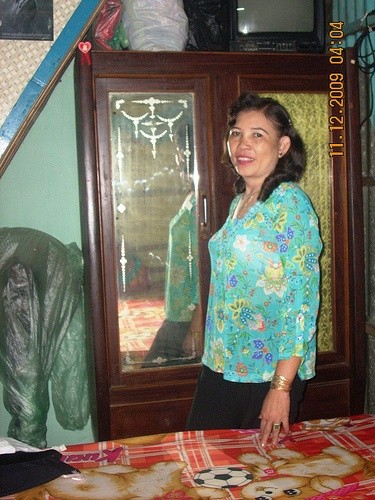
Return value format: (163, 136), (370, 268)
(228, 0), (326, 52)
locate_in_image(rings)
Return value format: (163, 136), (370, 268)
(273, 424), (280, 430)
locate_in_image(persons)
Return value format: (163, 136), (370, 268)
(141, 121), (204, 368)
(186, 94), (322, 447)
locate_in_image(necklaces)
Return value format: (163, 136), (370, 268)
(239, 189), (260, 208)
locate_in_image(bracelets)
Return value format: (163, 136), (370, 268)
(186, 327), (203, 338)
(270, 374), (292, 392)
